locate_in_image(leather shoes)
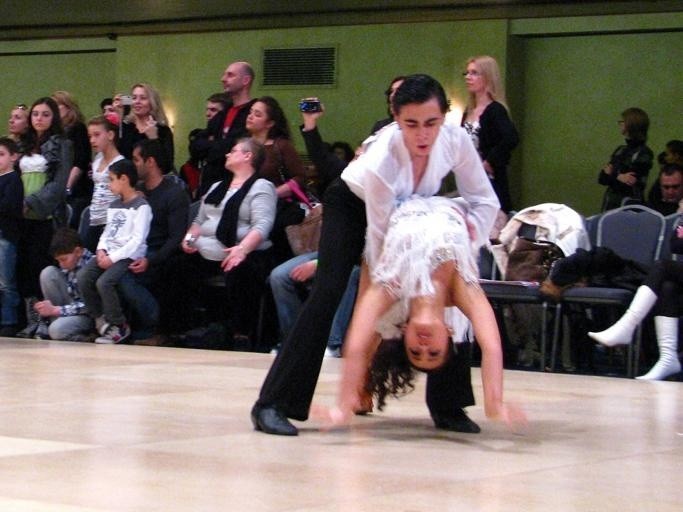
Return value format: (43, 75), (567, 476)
(432, 408), (479, 432)
(251, 408), (298, 435)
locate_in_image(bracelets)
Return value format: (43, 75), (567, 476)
(327, 405), (344, 424)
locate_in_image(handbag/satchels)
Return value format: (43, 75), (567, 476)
(505, 237), (565, 283)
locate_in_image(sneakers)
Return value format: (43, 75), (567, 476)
(95, 325), (130, 343)
(98, 320), (109, 336)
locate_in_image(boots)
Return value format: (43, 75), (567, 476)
(587, 284), (659, 347)
(17, 297), (50, 339)
(634, 316), (682, 381)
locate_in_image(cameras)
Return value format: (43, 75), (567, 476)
(299, 101), (320, 112)
(184, 234), (195, 247)
(120, 96), (132, 105)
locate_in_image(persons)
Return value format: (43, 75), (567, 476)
(598, 107), (654, 214)
(586, 201), (682, 381)
(647, 151), (667, 209)
(642, 163), (682, 216)
(1, 62), (361, 359)
(371, 54), (518, 279)
(665, 140), (682, 164)
(305, 195), (531, 434)
(251, 74), (500, 434)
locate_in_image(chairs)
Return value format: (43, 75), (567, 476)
(196, 196), (683, 380)
